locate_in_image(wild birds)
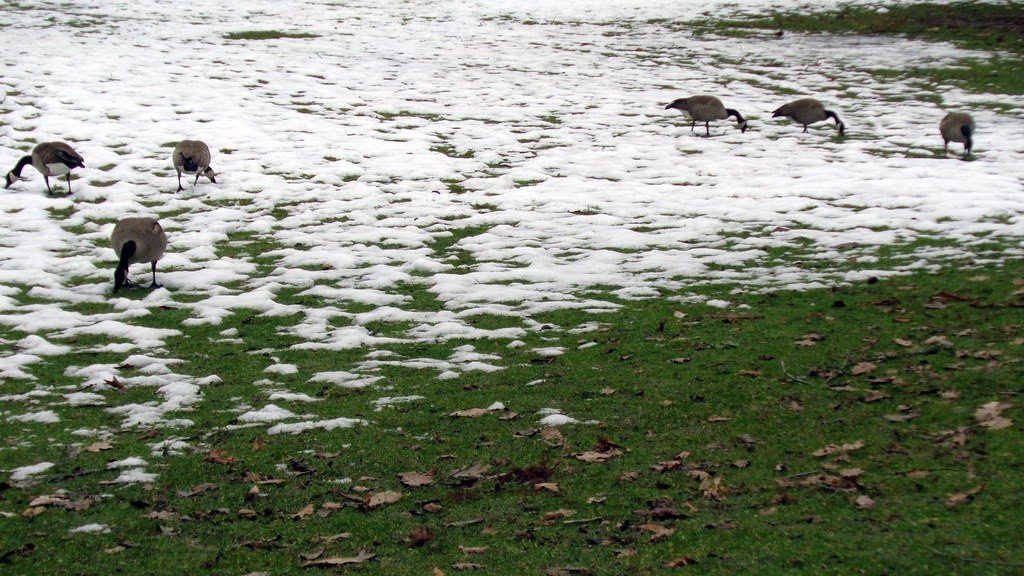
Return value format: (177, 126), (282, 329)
(665, 95), (746, 136)
(172, 140), (216, 192)
(4, 142), (85, 195)
(772, 98), (844, 134)
(939, 112), (975, 154)
(110, 216), (167, 292)
